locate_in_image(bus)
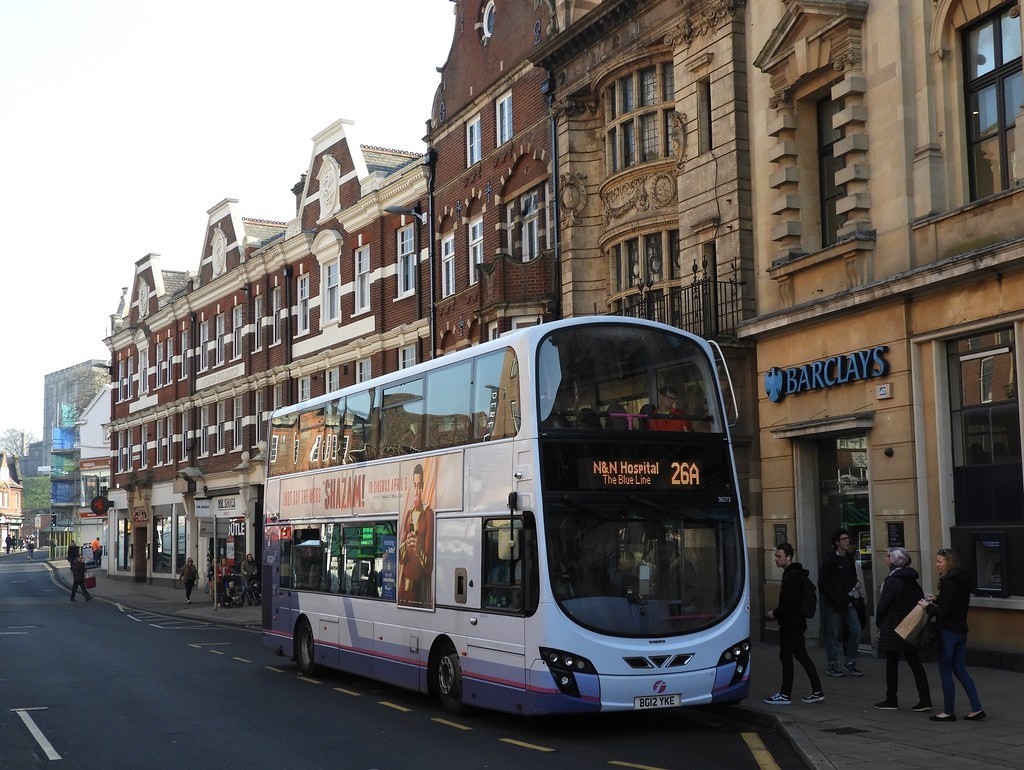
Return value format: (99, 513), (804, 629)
(261, 315), (754, 716)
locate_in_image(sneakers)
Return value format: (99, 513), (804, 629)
(909, 701), (933, 711)
(826, 664), (846, 677)
(843, 662), (863, 677)
(873, 701), (899, 709)
(763, 693), (792, 704)
(802, 691), (824, 703)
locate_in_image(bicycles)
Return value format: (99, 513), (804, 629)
(28, 549), (33, 563)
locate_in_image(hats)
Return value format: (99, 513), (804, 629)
(225, 569), (231, 573)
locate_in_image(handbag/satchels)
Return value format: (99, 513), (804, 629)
(85, 570), (96, 589)
(851, 591), (865, 608)
(894, 598), (941, 648)
(207, 564), (212, 577)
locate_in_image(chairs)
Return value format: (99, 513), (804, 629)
(576, 408), (602, 430)
(638, 403), (657, 431)
(605, 403), (629, 430)
(552, 408), (571, 428)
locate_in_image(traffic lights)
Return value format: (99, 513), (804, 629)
(51, 514), (57, 529)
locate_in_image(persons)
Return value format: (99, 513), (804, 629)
(763, 543), (824, 704)
(918, 548), (986, 721)
(207, 553), (261, 609)
(399, 464), (434, 609)
(90, 537), (101, 567)
(872, 547), (932, 711)
(559, 578), (577, 598)
(5, 534), (35, 562)
(648, 386), (693, 431)
(488, 540), (539, 610)
(70, 554), (93, 602)
(68, 540), (79, 569)
(659, 545), (698, 617)
(818, 528), (868, 677)
(177, 557), (199, 603)
(548, 530), (580, 570)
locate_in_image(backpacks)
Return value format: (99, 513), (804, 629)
(785, 565), (817, 618)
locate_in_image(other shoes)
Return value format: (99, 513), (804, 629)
(86, 596), (93, 601)
(185, 596), (187, 601)
(70, 598), (77, 601)
(963, 711), (987, 720)
(187, 600), (190, 604)
(218, 603), (221, 607)
(929, 714), (956, 721)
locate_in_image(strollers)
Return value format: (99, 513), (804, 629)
(221, 574), (243, 608)
(245, 573), (261, 607)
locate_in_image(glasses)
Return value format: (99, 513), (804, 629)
(188, 561), (192, 562)
(664, 395), (676, 402)
(840, 537), (851, 540)
